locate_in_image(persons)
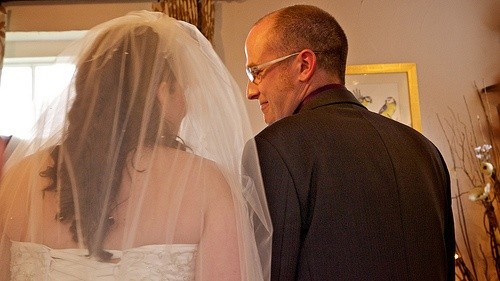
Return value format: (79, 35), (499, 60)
(0, 9), (272, 281)
(241, 5), (456, 281)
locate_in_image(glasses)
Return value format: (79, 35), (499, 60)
(245, 51), (319, 83)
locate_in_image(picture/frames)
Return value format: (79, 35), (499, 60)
(346, 62), (422, 132)
